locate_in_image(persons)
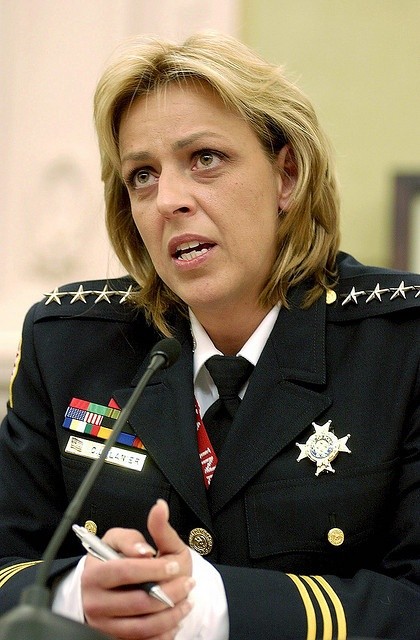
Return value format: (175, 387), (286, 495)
(0, 29), (420, 635)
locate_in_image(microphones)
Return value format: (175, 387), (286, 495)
(20, 337), (181, 608)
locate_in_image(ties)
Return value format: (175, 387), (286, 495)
(203, 359), (252, 456)
